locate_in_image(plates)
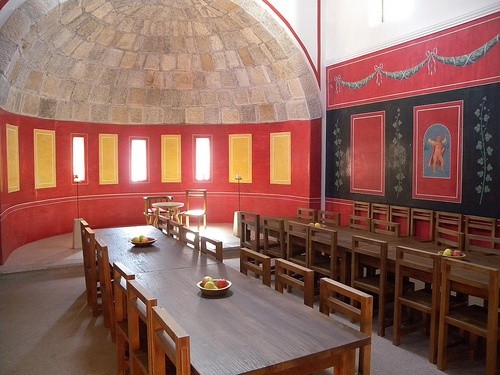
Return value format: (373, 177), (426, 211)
(128, 237), (157, 247)
(196, 278), (232, 295)
(437, 250), (466, 259)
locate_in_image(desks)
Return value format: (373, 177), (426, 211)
(93, 226), (371, 375)
(243, 217), (500, 294)
(151, 202), (184, 228)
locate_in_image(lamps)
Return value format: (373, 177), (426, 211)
(233, 176), (242, 237)
(73, 178), (82, 250)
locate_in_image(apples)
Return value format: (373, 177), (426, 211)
(202, 276), (228, 289)
(443, 249), (462, 256)
(309, 222), (321, 227)
(133, 236), (149, 242)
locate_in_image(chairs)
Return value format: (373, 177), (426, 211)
(143, 196), (173, 225)
(178, 190), (207, 229)
(81, 221), (191, 375)
(239, 202), (500, 375)
(157, 215), (223, 261)
